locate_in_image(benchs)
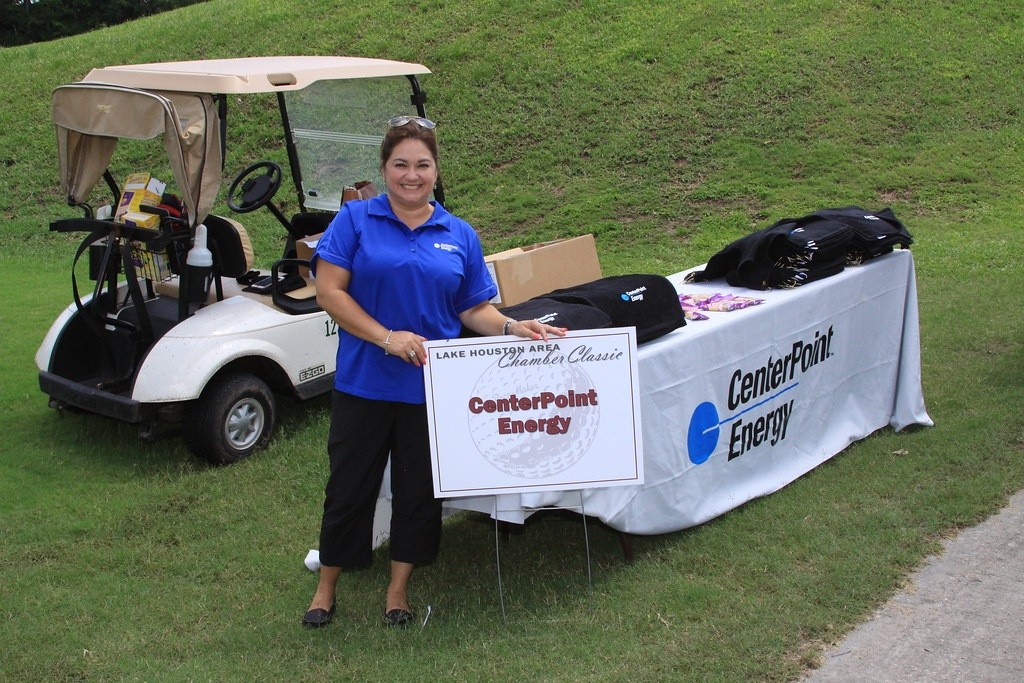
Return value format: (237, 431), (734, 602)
(155, 214), (317, 318)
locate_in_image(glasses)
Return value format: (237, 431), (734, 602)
(382, 115), (437, 156)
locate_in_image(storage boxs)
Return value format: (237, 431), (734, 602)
(482, 233), (603, 312)
(297, 232), (324, 278)
(113, 171), (170, 281)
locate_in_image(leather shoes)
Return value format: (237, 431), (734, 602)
(304, 593), (338, 629)
(384, 598), (414, 626)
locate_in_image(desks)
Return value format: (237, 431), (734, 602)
(440, 249), (935, 566)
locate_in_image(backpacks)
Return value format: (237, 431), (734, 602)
(456, 274), (686, 347)
(684, 205), (913, 291)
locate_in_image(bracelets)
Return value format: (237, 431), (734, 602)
(502, 319), (517, 336)
(383, 330), (392, 355)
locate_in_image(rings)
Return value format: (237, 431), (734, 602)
(409, 351), (416, 358)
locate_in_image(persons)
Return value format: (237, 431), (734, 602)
(302, 120), (567, 629)
